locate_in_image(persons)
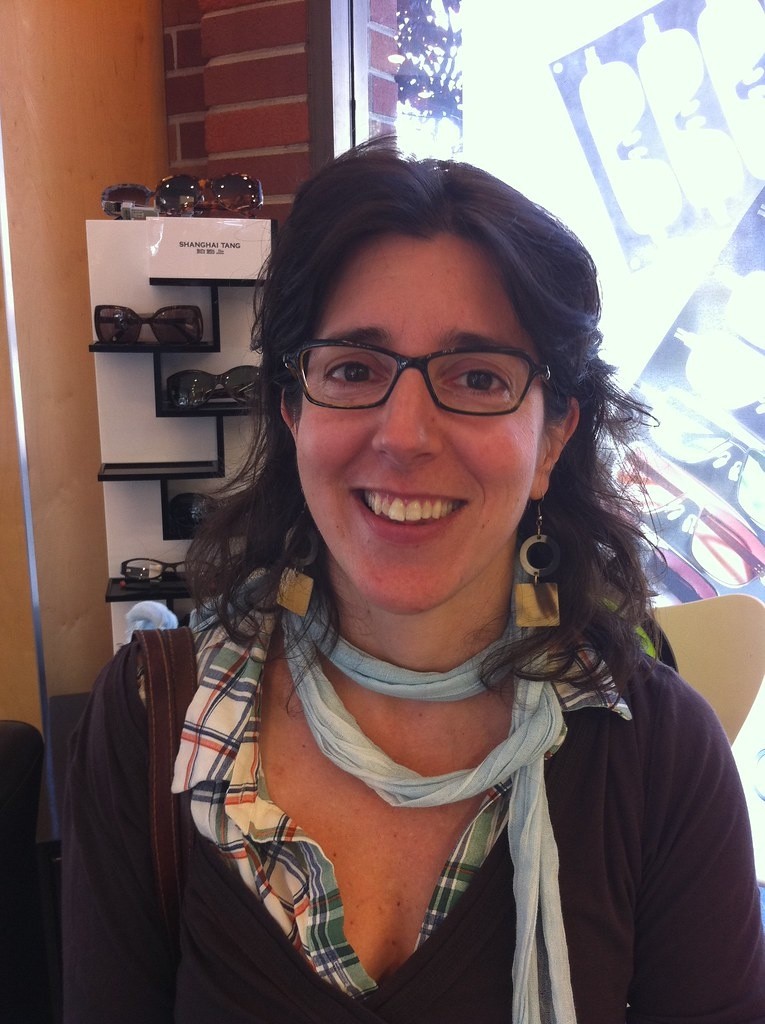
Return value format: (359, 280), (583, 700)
(59, 129), (765, 1024)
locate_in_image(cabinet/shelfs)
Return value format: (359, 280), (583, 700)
(85, 216), (277, 656)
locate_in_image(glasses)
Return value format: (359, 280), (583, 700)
(94, 304), (203, 347)
(168, 493), (219, 528)
(638, 381), (765, 531)
(674, 327), (765, 415)
(608, 438), (765, 590)
(166, 365), (264, 408)
(592, 487), (719, 610)
(100, 183), (208, 220)
(578, 45), (682, 243)
(283, 338), (551, 417)
(719, 266), (765, 350)
(156, 173), (264, 218)
(697, 0), (765, 181)
(121, 558), (217, 583)
(636, 13), (744, 218)
